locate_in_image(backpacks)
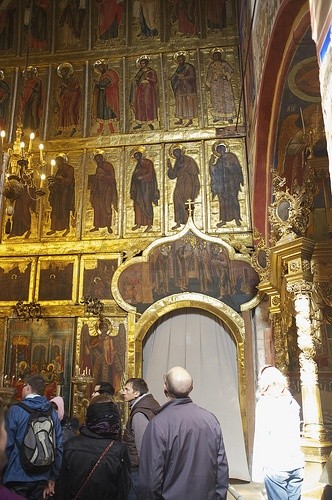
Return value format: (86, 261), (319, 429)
(14, 401), (57, 472)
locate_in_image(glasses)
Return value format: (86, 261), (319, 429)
(163, 374), (169, 392)
(92, 390), (105, 394)
(22, 383), (31, 389)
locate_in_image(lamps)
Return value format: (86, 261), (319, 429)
(0, 0), (59, 201)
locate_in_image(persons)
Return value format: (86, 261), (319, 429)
(91, 382), (114, 398)
(55, 394), (131, 500)
(0, 376), (80, 500)
(122, 378), (161, 500)
(252, 364), (305, 500)
(138, 366), (229, 500)
